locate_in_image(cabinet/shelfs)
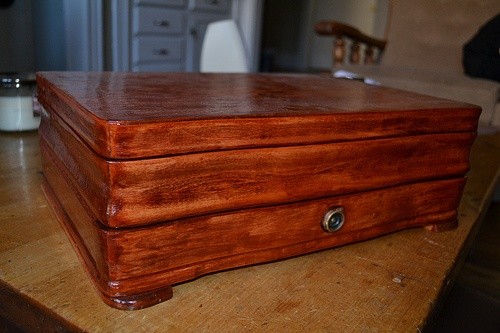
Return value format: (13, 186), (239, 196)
(130, 0), (231, 72)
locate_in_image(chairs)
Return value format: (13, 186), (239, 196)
(315, 21), (390, 73)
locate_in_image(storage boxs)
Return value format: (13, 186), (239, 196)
(33, 70), (482, 310)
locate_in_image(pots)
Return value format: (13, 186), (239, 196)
(0, 72), (41, 131)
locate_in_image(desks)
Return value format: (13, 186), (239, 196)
(0, 121), (499, 332)
